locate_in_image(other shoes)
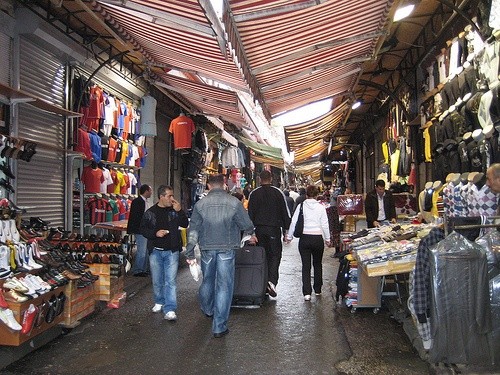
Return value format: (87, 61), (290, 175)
(214, 329), (228, 337)
(316, 292), (320, 296)
(0, 161), (15, 179)
(0, 178), (15, 193)
(0, 135), (38, 162)
(201, 309), (213, 320)
(134, 272), (148, 277)
(304, 295), (311, 300)
(266, 281), (277, 297)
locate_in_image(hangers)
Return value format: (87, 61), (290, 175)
(440, 217), (462, 240)
(179, 110), (185, 117)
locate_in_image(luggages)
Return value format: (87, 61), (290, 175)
(231, 231), (268, 308)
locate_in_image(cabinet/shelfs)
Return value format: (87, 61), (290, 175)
(37, 238), (126, 265)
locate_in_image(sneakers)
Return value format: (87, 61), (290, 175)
(164, 311), (177, 321)
(153, 303), (163, 312)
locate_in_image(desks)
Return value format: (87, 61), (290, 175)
(94, 221), (137, 256)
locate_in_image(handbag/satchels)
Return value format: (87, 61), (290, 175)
(189, 258), (201, 282)
(294, 202), (303, 237)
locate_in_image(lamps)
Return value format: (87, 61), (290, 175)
(350, 93), (362, 110)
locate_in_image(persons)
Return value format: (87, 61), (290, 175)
(485, 162), (500, 194)
(365, 179), (396, 229)
(247, 170), (333, 302)
(125, 183), (153, 278)
(140, 185), (189, 322)
(185, 174), (258, 339)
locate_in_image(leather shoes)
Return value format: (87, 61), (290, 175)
(0, 197), (129, 333)
